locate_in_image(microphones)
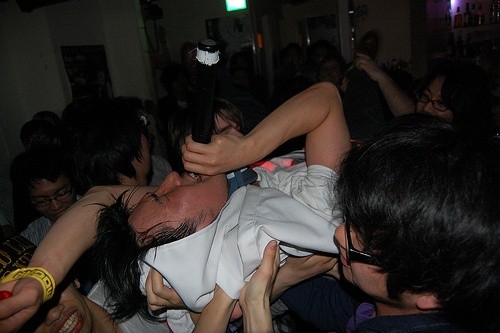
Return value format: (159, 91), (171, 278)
(191, 38), (217, 144)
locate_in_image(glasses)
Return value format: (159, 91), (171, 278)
(415, 91), (449, 112)
(345, 219), (392, 268)
(31, 183), (73, 208)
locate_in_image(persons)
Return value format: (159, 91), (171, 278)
(0, 29), (500, 333)
